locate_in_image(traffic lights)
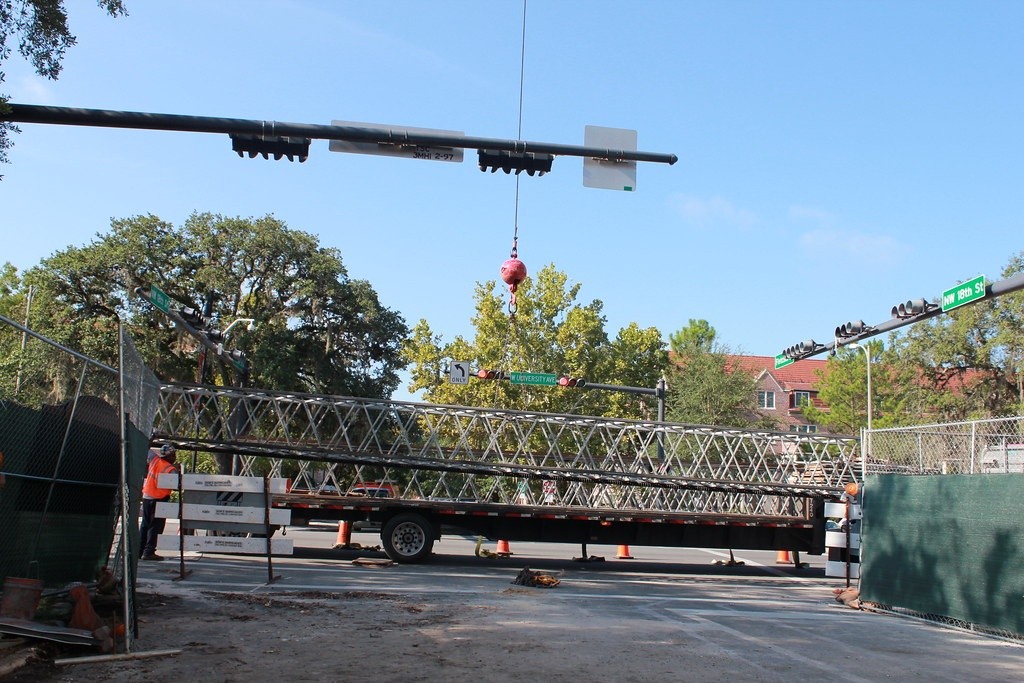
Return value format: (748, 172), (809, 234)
(782, 340), (817, 359)
(208, 328), (226, 343)
(178, 305), (206, 330)
(477, 369), (504, 380)
(559, 376), (585, 387)
(835, 320), (866, 339)
(891, 299), (929, 319)
(230, 349), (246, 361)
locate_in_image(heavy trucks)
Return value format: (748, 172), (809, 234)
(207, 443), (1023, 564)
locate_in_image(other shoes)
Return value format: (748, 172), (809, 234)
(142, 553), (164, 561)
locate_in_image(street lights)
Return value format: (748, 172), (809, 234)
(848, 343), (873, 458)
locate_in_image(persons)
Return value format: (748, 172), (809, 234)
(137, 445), (176, 560)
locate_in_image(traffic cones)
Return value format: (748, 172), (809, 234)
(335, 520), (348, 545)
(495, 540), (513, 554)
(776, 550), (792, 564)
(612, 545), (634, 558)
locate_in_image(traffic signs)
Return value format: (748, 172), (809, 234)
(774, 353), (795, 370)
(450, 360), (470, 384)
(150, 284), (170, 314)
(510, 372), (556, 385)
(941, 274), (985, 313)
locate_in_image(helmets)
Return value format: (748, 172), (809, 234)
(845, 483), (858, 498)
(159, 443), (178, 459)
